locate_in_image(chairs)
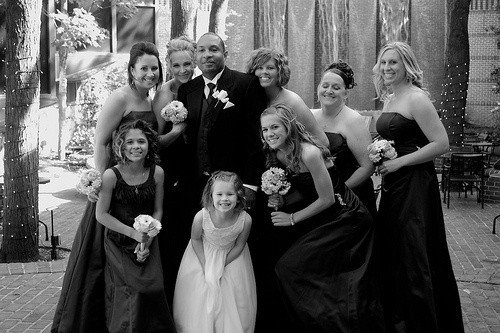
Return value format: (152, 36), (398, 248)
(447, 151), (487, 210)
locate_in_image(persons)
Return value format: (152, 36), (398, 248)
(95, 118), (175, 333)
(245, 48), (330, 151)
(259, 103), (387, 333)
(152, 35), (199, 151)
(49, 41), (164, 333)
(366, 41), (465, 333)
(303, 60), (379, 216)
(177, 32), (269, 177)
(172, 170), (258, 333)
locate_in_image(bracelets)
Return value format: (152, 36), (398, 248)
(290, 213), (295, 225)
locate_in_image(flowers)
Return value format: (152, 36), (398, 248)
(261, 167), (291, 213)
(213, 89), (231, 109)
(133, 214), (161, 251)
(161, 101), (188, 125)
(367, 138), (397, 176)
(76, 168), (104, 202)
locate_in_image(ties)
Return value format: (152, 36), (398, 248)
(205, 83), (216, 106)
